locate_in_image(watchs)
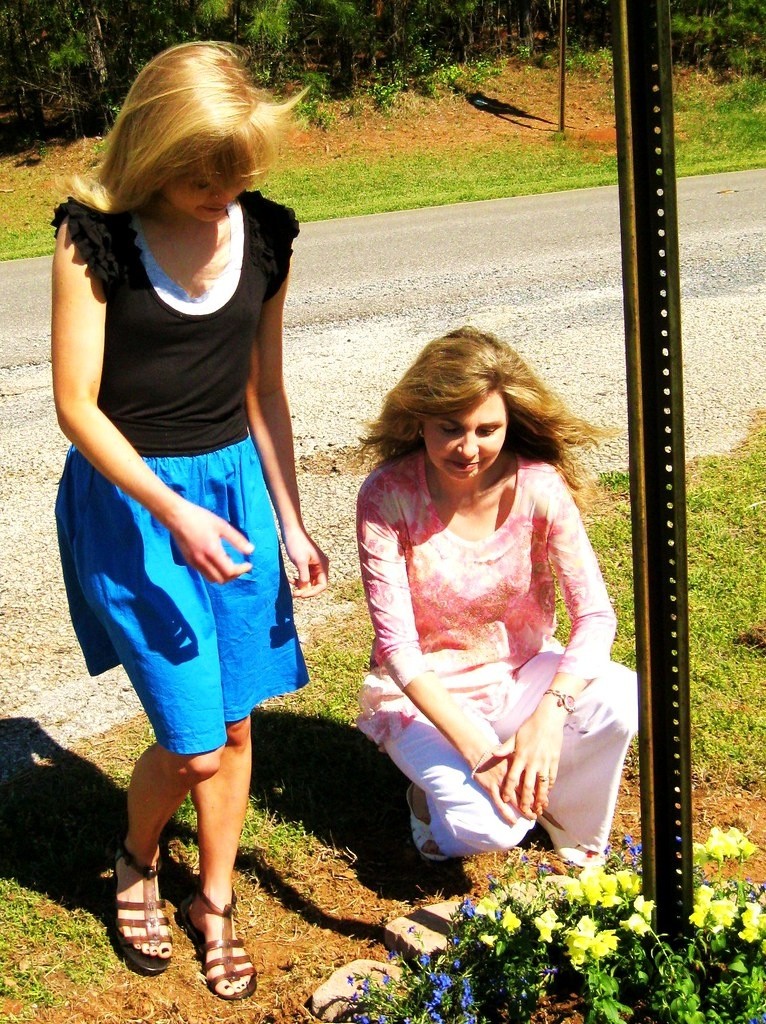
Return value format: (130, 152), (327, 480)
(543, 689), (576, 714)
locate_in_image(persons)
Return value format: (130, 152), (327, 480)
(50, 42), (329, 1000)
(352, 324), (637, 867)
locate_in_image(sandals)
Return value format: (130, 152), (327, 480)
(537, 811), (606, 868)
(177, 886), (257, 1001)
(407, 782), (450, 861)
(111, 836), (173, 973)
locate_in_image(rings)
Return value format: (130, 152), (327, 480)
(539, 776), (549, 782)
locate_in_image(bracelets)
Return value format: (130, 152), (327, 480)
(471, 748), (490, 781)
